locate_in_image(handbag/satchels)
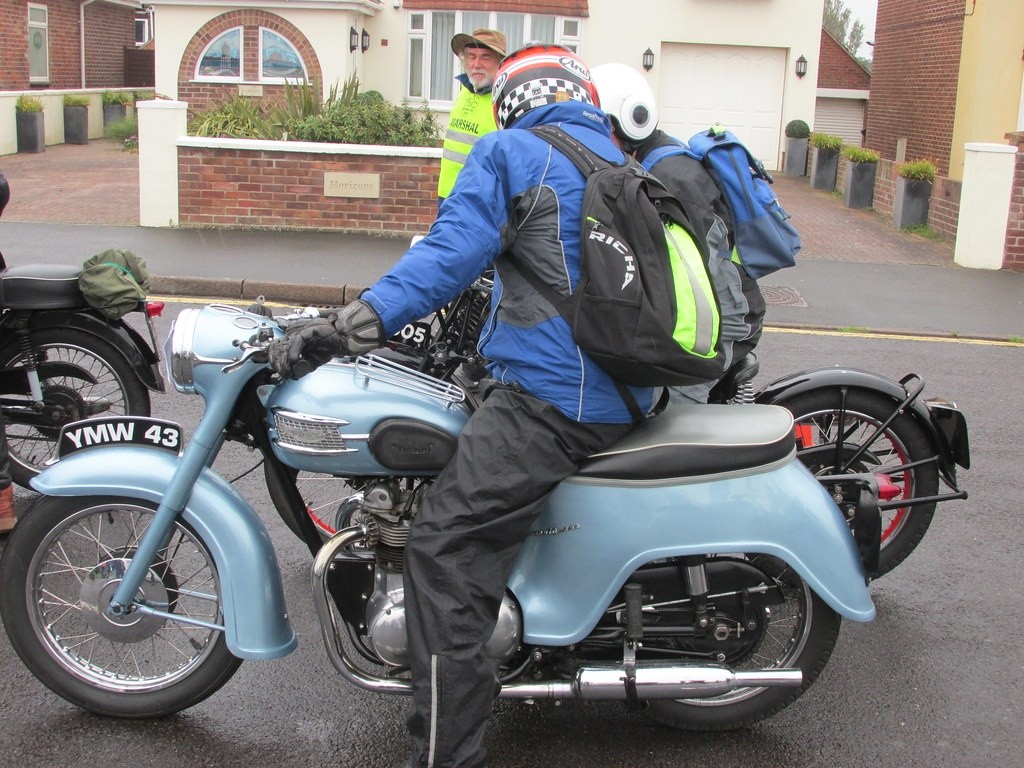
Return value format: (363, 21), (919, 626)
(79, 249), (151, 320)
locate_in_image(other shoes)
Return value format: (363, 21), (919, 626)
(0, 482), (16, 531)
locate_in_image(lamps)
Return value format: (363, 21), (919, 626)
(349, 26), (359, 53)
(362, 28), (370, 53)
(643, 47), (654, 73)
(796, 54), (807, 78)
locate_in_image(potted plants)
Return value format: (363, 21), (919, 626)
(838, 142), (879, 209)
(15, 93), (47, 152)
(63, 94), (90, 144)
(104, 90), (126, 135)
(784, 119), (809, 176)
(809, 131), (841, 188)
(892, 159), (941, 231)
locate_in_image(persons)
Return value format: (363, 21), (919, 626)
(589, 62), (767, 403)
(438, 27), (510, 212)
(0, 174), (17, 534)
(266, 42), (668, 768)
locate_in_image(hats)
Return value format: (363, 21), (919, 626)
(451, 29), (506, 56)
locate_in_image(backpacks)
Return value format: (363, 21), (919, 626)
(640, 128), (802, 279)
(505, 124), (726, 422)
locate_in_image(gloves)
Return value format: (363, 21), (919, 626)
(268, 298), (382, 381)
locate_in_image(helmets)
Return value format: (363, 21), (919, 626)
(492, 43), (600, 129)
(590, 63), (660, 153)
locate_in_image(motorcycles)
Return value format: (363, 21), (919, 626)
(273, 270), (971, 587)
(0, 275), (876, 729)
(0, 171), (167, 495)
